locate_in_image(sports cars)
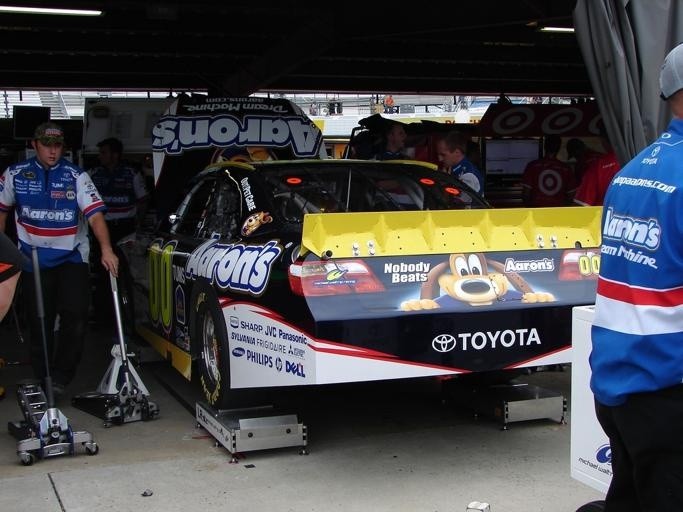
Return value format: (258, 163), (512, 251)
(116, 88), (605, 409)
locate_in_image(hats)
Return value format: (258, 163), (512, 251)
(34, 122), (67, 147)
(659, 42), (683, 100)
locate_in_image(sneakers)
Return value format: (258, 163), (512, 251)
(45, 381), (65, 398)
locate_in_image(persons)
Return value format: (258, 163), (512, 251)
(565, 138), (621, 206)
(516, 132), (583, 208)
(0, 118), (122, 407)
(0, 226), (32, 328)
(84, 134), (148, 337)
(574, 38), (680, 509)
(437, 120), (486, 207)
(576, 148), (621, 205)
(376, 122), (424, 207)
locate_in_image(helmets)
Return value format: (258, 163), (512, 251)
(566, 137), (583, 160)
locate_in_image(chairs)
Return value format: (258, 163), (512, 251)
(275, 166), (429, 232)
